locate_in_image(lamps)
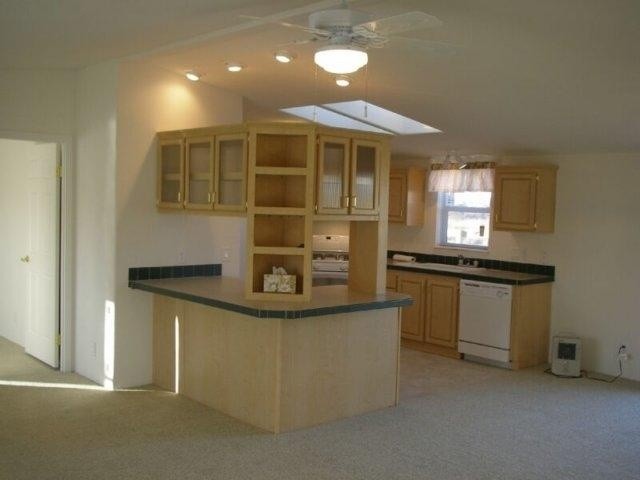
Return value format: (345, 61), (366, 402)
(312, 45), (368, 75)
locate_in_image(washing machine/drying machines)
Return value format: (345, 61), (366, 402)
(456, 278), (513, 370)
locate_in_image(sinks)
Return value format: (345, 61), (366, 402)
(415, 263), (488, 272)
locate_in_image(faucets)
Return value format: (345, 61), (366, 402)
(458, 254), (464, 267)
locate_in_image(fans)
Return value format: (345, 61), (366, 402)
(239, 1), (465, 50)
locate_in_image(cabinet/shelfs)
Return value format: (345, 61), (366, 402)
(157, 122), (247, 216)
(248, 124), (314, 302)
(493, 164), (559, 233)
(389, 166), (427, 227)
(387, 270), (463, 360)
(315, 126), (382, 220)
